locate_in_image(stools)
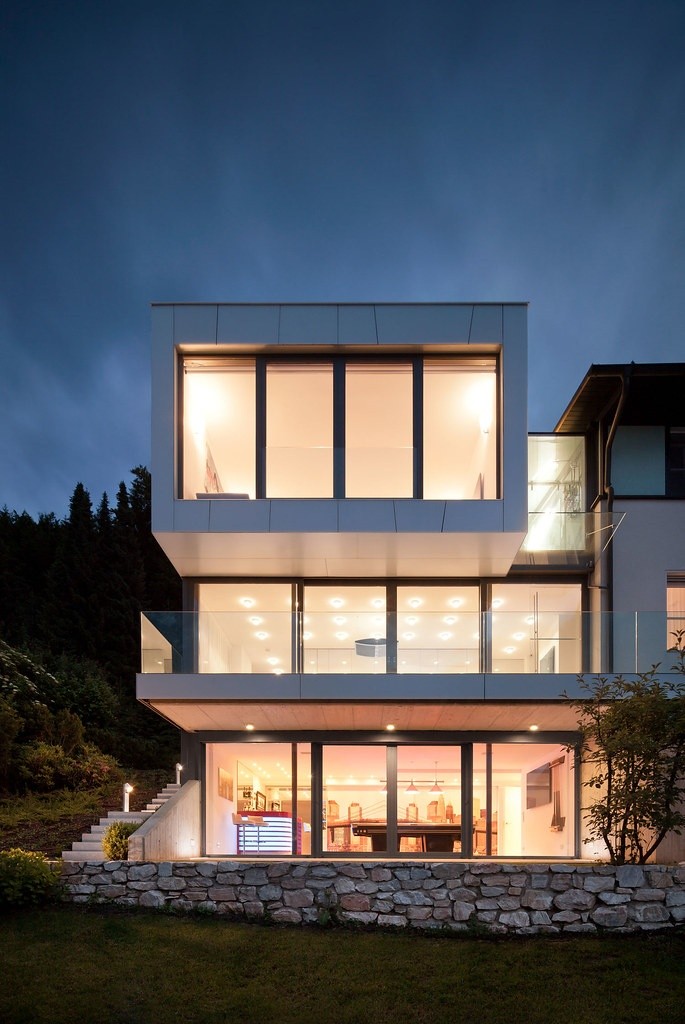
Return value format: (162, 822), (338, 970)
(233, 812), (269, 854)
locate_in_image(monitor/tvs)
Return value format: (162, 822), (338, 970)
(526, 762), (552, 809)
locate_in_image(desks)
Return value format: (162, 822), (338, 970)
(351, 823), (475, 853)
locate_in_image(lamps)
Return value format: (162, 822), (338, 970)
(405, 782), (419, 792)
(430, 762), (443, 792)
